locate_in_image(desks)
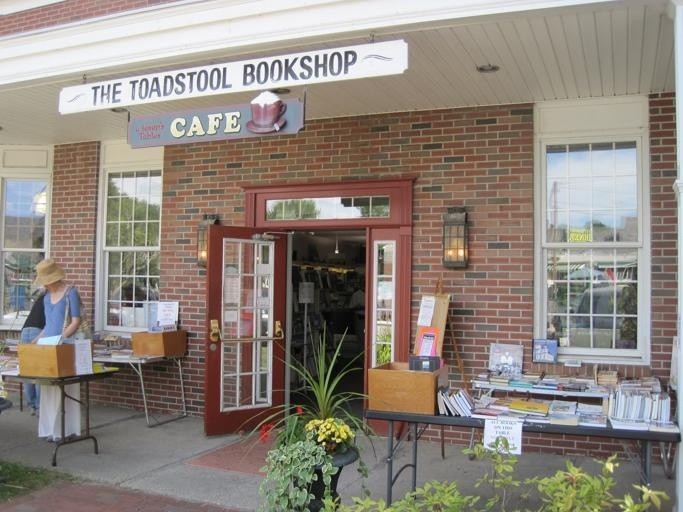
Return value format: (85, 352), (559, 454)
(469, 374), (670, 480)
(367, 397), (681, 510)
(0, 343), (187, 466)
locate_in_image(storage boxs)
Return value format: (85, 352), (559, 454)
(132, 331), (188, 358)
(366, 361), (450, 416)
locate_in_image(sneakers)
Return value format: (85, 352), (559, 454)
(47, 432), (76, 443)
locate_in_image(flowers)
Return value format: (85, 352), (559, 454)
(232, 320), (384, 468)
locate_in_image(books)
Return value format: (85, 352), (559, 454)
(407, 339), (680, 433)
(0, 323), (175, 373)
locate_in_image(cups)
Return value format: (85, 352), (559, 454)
(250, 101), (287, 127)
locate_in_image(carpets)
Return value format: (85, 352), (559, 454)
(181, 428), (284, 477)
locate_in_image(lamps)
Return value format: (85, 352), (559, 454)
(197, 214), (218, 268)
(298, 282), (315, 396)
(442, 206), (469, 269)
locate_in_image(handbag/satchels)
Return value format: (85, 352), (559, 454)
(60, 286), (93, 346)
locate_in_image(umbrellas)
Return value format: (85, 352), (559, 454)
(564, 266), (605, 288)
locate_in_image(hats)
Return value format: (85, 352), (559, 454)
(30, 257), (68, 289)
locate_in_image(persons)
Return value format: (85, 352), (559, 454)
(347, 277), (365, 376)
(18, 293), (46, 418)
(29, 259), (82, 443)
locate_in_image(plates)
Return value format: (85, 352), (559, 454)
(246, 118), (287, 133)
